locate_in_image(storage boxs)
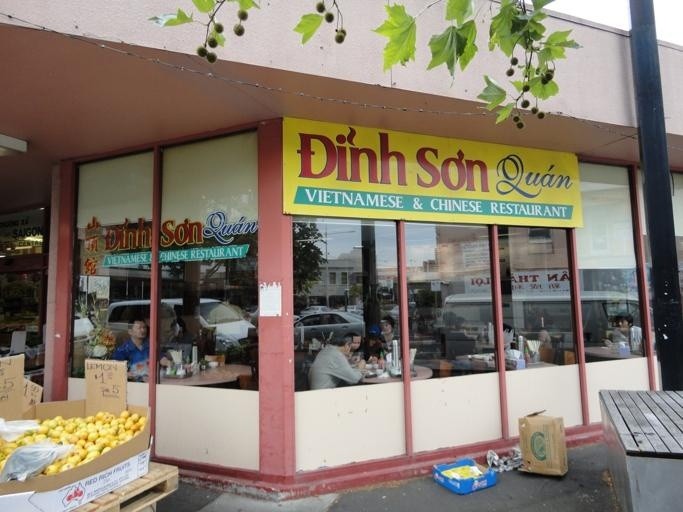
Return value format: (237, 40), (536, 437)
(516, 409), (569, 479)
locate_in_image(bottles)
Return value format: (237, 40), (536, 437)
(516, 353), (525, 369)
(377, 352), (385, 369)
(524, 347), (530, 361)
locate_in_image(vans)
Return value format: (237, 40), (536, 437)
(433, 291), (656, 355)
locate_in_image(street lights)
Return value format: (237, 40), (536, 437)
(324, 230), (357, 308)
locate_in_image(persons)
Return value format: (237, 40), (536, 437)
(306, 311), (556, 390)
(603, 313), (643, 352)
(109, 317), (197, 381)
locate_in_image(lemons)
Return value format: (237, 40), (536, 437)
(0, 410), (147, 477)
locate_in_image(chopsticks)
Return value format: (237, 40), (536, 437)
(167, 348), (183, 364)
(526, 340), (541, 352)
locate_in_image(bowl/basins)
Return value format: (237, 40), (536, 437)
(208, 361), (218, 368)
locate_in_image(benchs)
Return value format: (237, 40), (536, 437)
(597, 387), (681, 511)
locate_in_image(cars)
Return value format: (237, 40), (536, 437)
(104, 298), (256, 365)
(294, 302), (416, 353)
